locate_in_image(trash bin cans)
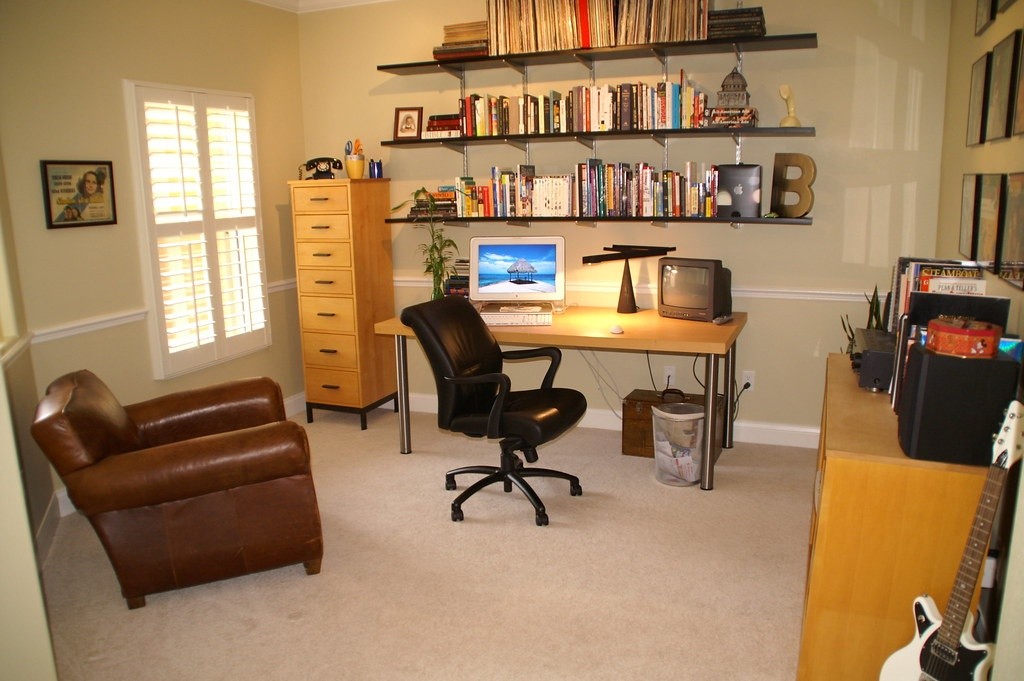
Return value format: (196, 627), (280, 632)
(652, 402), (704, 488)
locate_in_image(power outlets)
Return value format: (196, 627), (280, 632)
(662, 366), (677, 390)
(742, 369), (755, 393)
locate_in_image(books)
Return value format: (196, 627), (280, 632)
(433, 0), (767, 61)
(421, 68), (759, 140)
(408, 158), (719, 220)
(887, 256), (1024, 415)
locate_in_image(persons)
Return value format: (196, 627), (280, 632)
(401, 117), (415, 132)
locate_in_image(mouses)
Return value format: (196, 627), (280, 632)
(611, 325), (624, 334)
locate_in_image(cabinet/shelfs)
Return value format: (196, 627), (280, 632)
(377, 33), (816, 223)
(794, 356), (993, 680)
(289, 177), (397, 430)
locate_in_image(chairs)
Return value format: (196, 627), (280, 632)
(400, 293), (588, 526)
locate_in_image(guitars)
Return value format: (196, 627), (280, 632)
(878, 400), (1024, 681)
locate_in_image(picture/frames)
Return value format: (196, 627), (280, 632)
(39, 159), (117, 230)
(392, 106), (421, 144)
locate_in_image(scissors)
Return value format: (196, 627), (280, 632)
(354, 140), (360, 156)
(345, 141), (353, 156)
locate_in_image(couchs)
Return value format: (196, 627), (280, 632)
(27, 367), (325, 612)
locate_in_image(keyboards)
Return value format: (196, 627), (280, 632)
(480, 313), (553, 326)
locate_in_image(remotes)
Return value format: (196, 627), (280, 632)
(712, 315), (734, 325)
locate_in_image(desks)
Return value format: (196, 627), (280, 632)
(373, 307), (747, 491)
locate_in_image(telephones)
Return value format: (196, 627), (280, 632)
(304, 156), (343, 179)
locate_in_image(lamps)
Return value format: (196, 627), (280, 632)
(582, 243), (678, 316)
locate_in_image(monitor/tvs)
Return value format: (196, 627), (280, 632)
(468, 236), (566, 314)
(657, 257), (732, 323)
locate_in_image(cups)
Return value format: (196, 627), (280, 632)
(370, 163), (382, 179)
(344, 155), (365, 179)
